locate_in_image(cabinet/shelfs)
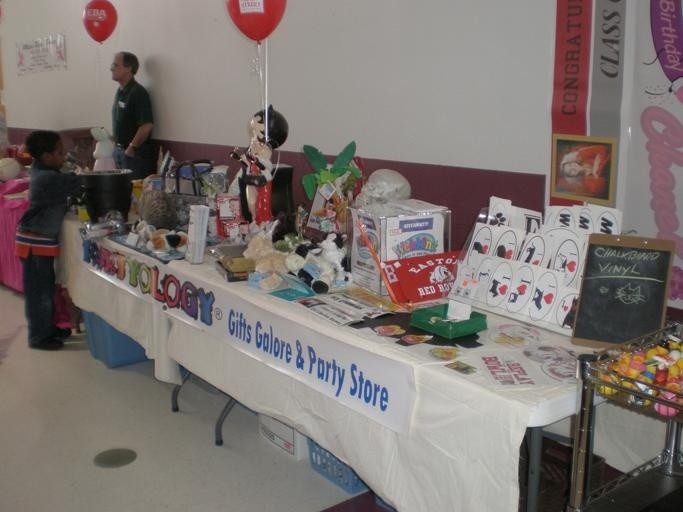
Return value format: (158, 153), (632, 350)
(564, 320), (682, 511)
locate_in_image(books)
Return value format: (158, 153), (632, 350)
(187, 202), (210, 267)
(213, 255), (258, 283)
(378, 249), (466, 308)
(214, 245), (254, 273)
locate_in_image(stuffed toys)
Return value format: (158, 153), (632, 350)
(240, 211), (348, 296)
(127, 189), (189, 254)
(598, 339), (683, 416)
(89, 128), (116, 172)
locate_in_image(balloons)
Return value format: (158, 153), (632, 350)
(225, 0), (289, 44)
(80, 0), (119, 46)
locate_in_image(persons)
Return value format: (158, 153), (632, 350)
(12, 130), (95, 350)
(225, 101), (289, 226)
(110, 51), (162, 180)
(557, 144), (610, 197)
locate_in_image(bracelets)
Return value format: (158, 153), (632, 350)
(128, 143), (138, 150)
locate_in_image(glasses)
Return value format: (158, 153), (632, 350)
(110, 63), (124, 67)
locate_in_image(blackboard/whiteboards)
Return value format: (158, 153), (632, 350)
(571, 233), (676, 351)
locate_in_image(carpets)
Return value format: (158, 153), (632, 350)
(321, 436), (624, 511)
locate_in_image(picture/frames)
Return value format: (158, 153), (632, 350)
(551, 133), (618, 208)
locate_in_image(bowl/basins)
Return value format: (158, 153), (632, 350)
(80, 168), (133, 223)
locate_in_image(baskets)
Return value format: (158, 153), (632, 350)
(306, 439), (368, 495)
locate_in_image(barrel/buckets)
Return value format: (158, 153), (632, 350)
(75, 168), (134, 222)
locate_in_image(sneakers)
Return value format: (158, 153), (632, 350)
(29, 337), (64, 350)
(53, 326), (71, 339)
